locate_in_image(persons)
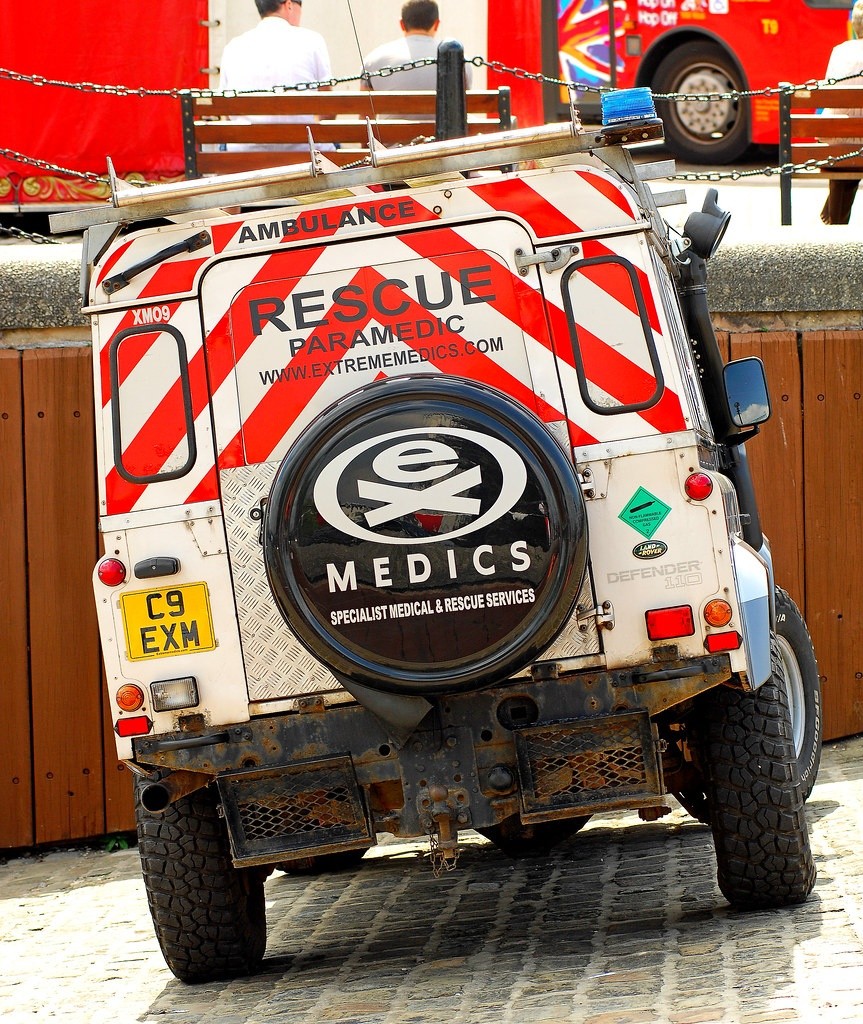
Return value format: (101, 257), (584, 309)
(821, 0), (863, 224)
(218, 0), (341, 152)
(358, 0), (472, 147)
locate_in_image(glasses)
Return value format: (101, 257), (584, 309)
(281, 0), (301, 7)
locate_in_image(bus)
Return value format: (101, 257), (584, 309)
(556, 0), (863, 164)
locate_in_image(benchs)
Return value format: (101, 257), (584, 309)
(179, 87), (511, 182)
(779, 83), (863, 226)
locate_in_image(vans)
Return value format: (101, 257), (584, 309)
(46, 123), (824, 981)
